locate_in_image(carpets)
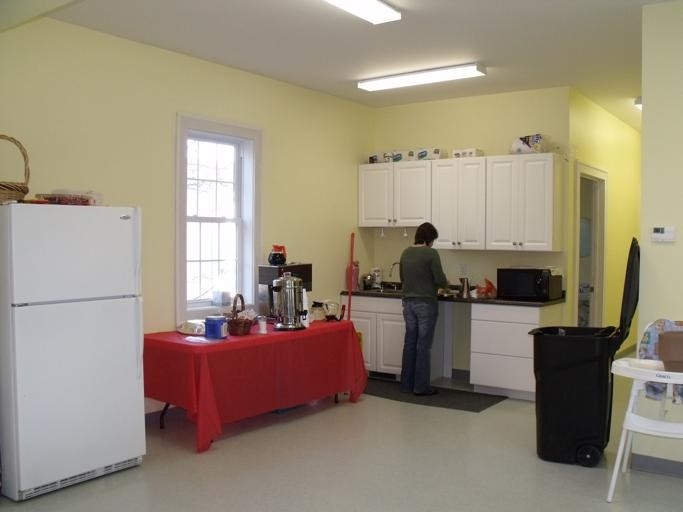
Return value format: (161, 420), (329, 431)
(364, 378), (509, 413)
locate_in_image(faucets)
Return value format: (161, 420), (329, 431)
(388, 262), (400, 278)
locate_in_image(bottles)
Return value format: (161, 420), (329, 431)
(205, 315), (231, 340)
(363, 272), (373, 290)
(462, 279), (468, 299)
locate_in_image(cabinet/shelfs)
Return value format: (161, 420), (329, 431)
(486, 151), (565, 252)
(432, 157), (486, 250)
(340, 295), (444, 382)
(469, 303), (562, 402)
(358, 160), (432, 228)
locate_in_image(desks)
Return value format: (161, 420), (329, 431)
(143, 318), (368, 452)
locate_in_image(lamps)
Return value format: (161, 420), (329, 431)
(357, 64), (487, 92)
(326, 0), (402, 25)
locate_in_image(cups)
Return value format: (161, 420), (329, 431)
(257, 315), (268, 334)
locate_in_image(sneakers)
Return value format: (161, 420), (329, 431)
(404, 387), (437, 396)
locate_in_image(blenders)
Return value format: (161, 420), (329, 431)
(271, 272), (306, 331)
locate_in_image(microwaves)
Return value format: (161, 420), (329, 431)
(496, 268), (563, 302)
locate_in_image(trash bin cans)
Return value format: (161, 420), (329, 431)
(527, 236), (641, 467)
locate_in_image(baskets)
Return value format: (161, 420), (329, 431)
(0, 135), (29, 204)
(228, 294), (252, 335)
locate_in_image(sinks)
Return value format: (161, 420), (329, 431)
(377, 288), (403, 294)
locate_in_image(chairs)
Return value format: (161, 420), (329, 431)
(605, 319), (683, 503)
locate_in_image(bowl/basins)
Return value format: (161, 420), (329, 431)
(177, 319), (210, 335)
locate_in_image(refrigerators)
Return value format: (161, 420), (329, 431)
(0, 203), (147, 502)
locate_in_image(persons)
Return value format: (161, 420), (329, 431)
(399, 223), (448, 396)
